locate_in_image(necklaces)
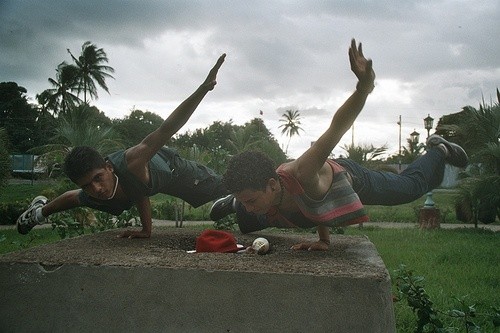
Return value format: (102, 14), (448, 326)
(274, 175), (285, 208)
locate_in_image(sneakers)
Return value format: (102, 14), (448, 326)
(209, 192), (238, 221)
(426, 135), (469, 168)
(16, 196), (51, 236)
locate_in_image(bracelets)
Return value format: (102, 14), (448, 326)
(355, 80), (373, 96)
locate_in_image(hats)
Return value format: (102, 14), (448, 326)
(186, 229), (237, 254)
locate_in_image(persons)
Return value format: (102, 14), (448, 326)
(15, 52), (233, 241)
(208, 38), (469, 252)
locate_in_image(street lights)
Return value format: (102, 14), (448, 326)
(419, 113), (441, 226)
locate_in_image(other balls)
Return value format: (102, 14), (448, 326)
(252, 237), (270, 255)
